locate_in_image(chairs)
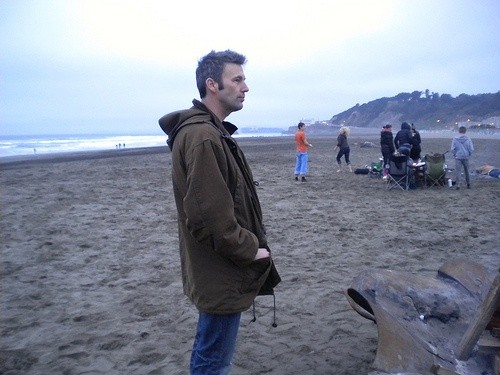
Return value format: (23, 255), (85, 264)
(424, 153), (448, 188)
(387, 154), (415, 192)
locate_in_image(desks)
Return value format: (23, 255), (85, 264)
(412, 165), (426, 172)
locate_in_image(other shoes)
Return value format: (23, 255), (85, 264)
(295, 176), (298, 181)
(302, 177), (307, 182)
(466, 184), (470, 189)
(455, 186), (460, 190)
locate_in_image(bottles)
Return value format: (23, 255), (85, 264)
(448, 178), (452, 187)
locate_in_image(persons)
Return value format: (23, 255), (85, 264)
(451, 125), (475, 190)
(380, 124), (395, 170)
(158, 48), (281, 374)
(333, 126), (354, 172)
(295, 122), (313, 183)
(393, 121), (422, 188)
